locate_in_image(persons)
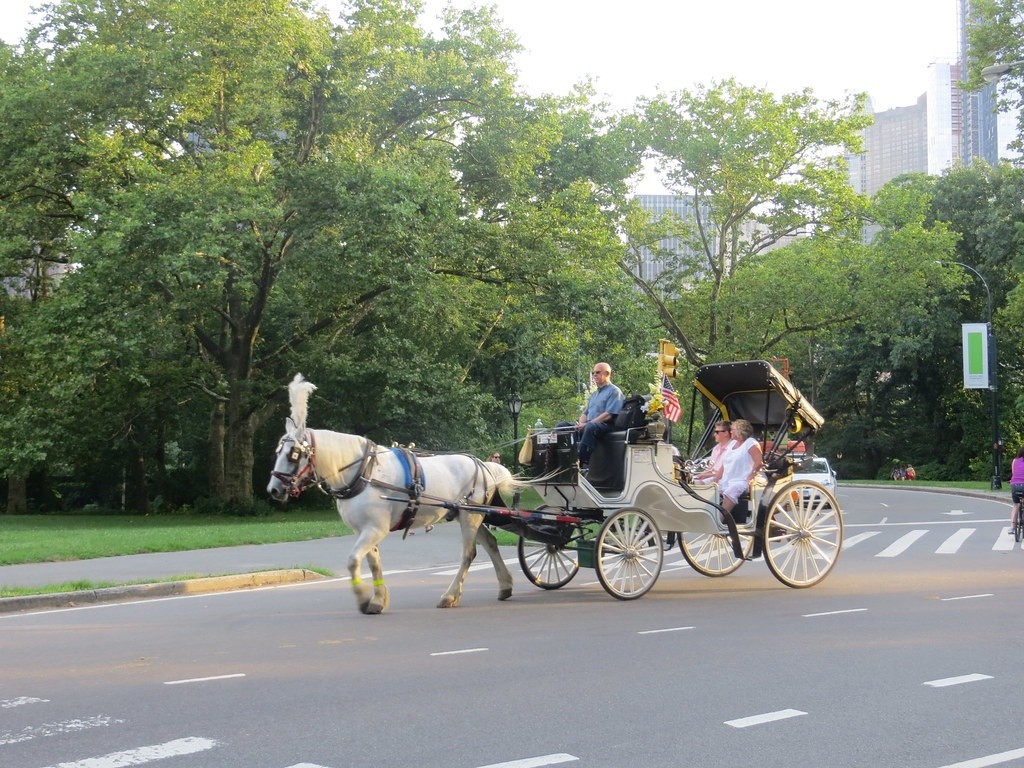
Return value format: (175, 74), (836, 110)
(693, 420), (763, 523)
(893, 464), (915, 481)
(1008, 446), (1024, 534)
(548, 362), (625, 482)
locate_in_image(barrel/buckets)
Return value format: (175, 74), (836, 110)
(575, 539), (595, 568)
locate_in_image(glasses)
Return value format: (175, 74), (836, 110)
(592, 371), (601, 374)
(714, 429), (725, 434)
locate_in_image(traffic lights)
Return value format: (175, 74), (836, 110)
(658, 354), (677, 377)
(663, 343), (680, 379)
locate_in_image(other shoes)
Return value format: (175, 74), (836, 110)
(582, 460), (589, 469)
(1008, 527), (1015, 534)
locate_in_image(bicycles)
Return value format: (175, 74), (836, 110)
(1008, 492), (1024, 542)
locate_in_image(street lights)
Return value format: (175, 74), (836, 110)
(930, 261), (1004, 492)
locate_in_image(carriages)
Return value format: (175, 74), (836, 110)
(266, 357), (844, 615)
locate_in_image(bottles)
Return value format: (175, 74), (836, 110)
(534, 419), (543, 434)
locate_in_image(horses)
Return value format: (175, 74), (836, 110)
(266, 417), (515, 614)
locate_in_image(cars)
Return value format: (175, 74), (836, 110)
(792, 457), (837, 509)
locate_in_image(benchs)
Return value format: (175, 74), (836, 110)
(533, 399), (648, 487)
(721, 454), (795, 523)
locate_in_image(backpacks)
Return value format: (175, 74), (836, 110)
(614, 394), (646, 430)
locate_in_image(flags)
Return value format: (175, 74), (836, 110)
(662, 375), (683, 423)
